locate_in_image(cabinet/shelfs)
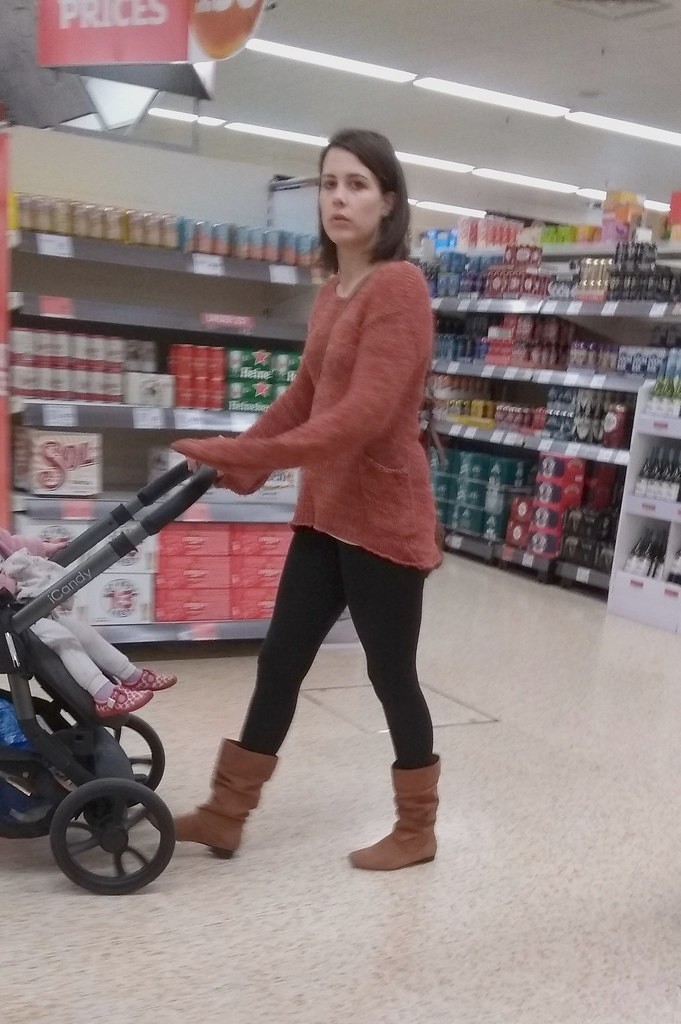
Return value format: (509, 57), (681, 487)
(605, 377), (681, 634)
(6, 226), (355, 645)
(407, 246), (681, 591)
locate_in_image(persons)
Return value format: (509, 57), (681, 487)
(147, 128), (446, 870)
(0, 526), (177, 718)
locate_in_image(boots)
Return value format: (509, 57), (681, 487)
(348, 754), (441, 870)
(145, 738), (278, 859)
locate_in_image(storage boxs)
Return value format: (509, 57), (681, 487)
(145, 443), (297, 503)
(13, 515), (294, 622)
(14, 425), (104, 499)
(427, 445), (615, 577)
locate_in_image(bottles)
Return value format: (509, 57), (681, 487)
(623, 374), (681, 588)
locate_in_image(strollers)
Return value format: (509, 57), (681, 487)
(0, 458), (216, 897)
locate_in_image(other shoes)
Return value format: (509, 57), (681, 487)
(94, 685), (154, 717)
(122, 669), (177, 692)
